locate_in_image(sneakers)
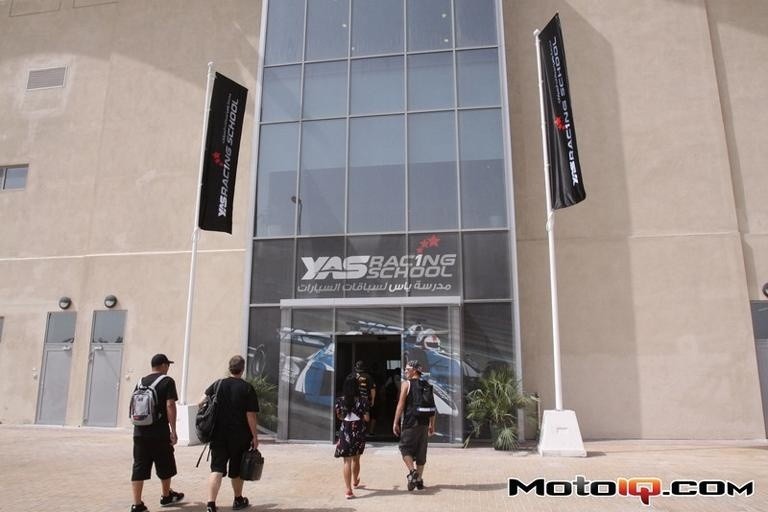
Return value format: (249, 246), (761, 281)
(345, 490), (354, 499)
(130, 502), (148, 512)
(159, 488), (184, 506)
(353, 476), (360, 488)
(206, 502), (217, 511)
(406, 469), (419, 491)
(417, 478), (423, 490)
(233, 495), (249, 510)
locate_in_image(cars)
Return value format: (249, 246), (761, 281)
(245, 317), (493, 437)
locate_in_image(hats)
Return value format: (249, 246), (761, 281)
(151, 354), (175, 367)
(354, 361), (371, 372)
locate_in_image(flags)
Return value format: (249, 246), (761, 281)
(536, 11), (589, 211)
(198, 70), (250, 237)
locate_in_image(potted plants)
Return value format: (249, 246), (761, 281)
(461, 365), (541, 457)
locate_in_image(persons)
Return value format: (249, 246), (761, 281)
(332, 373), (371, 498)
(394, 360), (437, 490)
(129, 353), (184, 511)
(423, 335), (441, 352)
(197, 355), (260, 511)
(348, 360), (401, 438)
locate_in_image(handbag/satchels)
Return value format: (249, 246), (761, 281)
(238, 446), (264, 481)
(195, 380), (222, 444)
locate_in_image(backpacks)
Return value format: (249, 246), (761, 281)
(128, 374), (166, 427)
(408, 378), (436, 417)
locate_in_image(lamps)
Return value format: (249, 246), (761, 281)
(59, 297), (71, 309)
(104, 295), (117, 307)
